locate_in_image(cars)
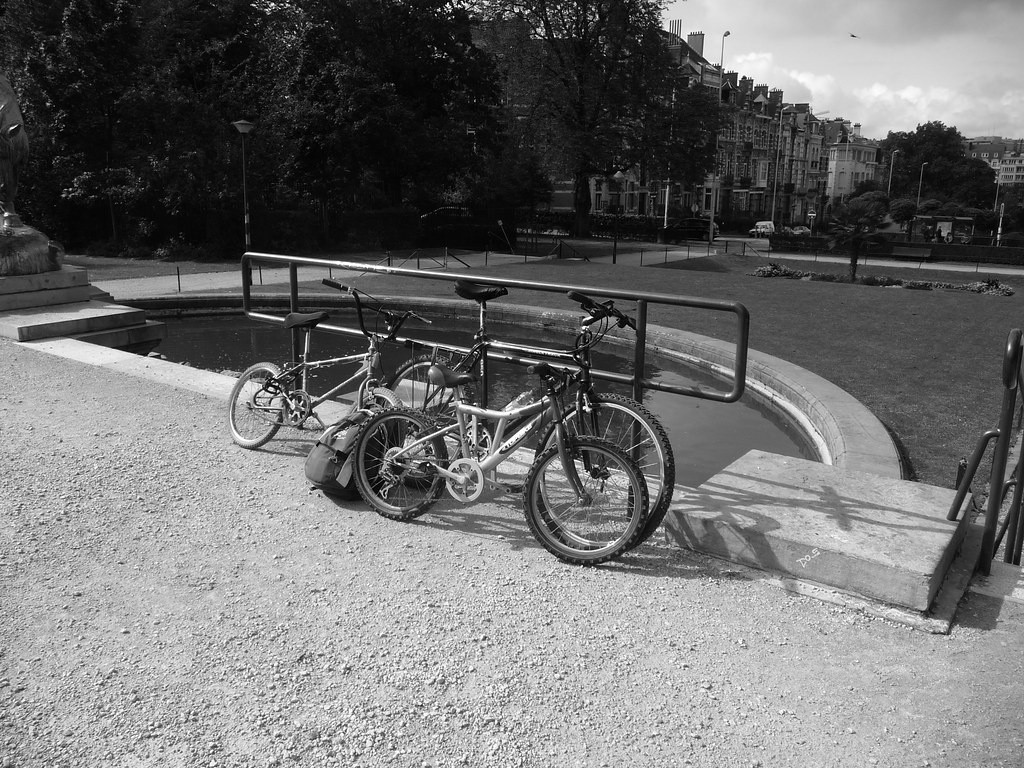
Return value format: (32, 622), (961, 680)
(421, 207), (471, 220)
(664, 218), (720, 243)
(792, 226), (812, 236)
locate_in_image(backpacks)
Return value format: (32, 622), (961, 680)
(304, 403), (400, 502)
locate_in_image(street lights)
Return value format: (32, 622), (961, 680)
(233, 119), (256, 252)
(917, 162), (929, 208)
(708, 31), (730, 244)
(772, 105), (792, 222)
(888, 150), (900, 198)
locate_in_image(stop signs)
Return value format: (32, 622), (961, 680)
(808, 210), (816, 220)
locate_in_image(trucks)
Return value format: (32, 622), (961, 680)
(749, 222), (775, 239)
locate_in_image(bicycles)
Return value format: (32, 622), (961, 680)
(381, 279), (675, 557)
(225, 278), (431, 493)
(351, 361), (649, 566)
